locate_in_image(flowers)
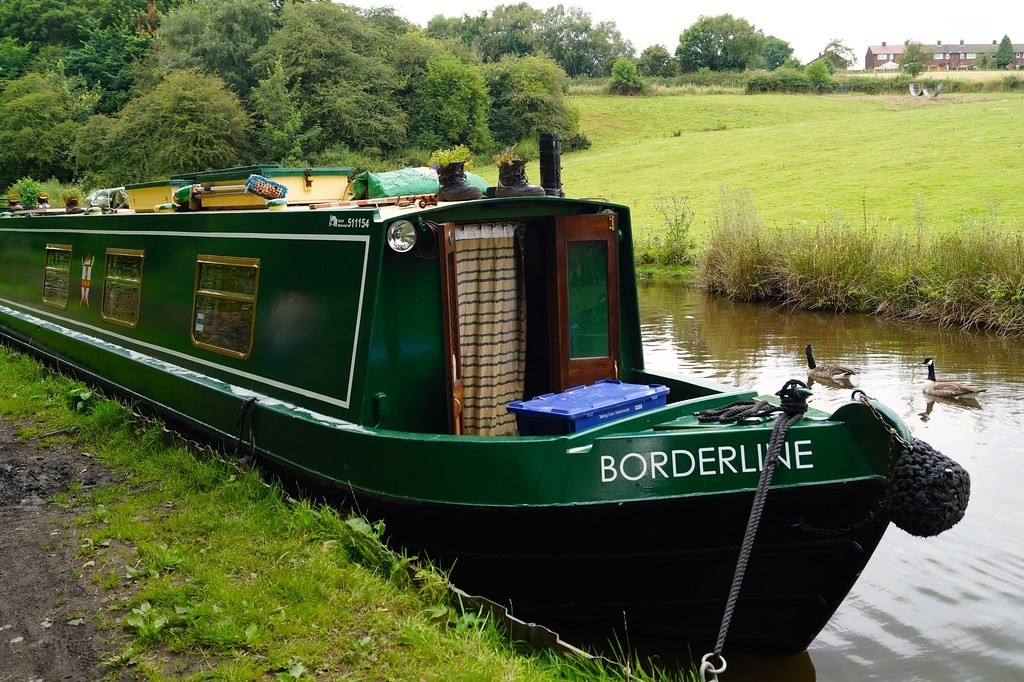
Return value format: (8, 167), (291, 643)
(427, 143), (471, 171)
(492, 143), (521, 170)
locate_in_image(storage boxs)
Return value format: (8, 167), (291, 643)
(506, 378), (670, 437)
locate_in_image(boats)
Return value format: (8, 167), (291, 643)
(1, 130), (973, 676)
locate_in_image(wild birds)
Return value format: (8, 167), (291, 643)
(804, 343), (856, 381)
(917, 357), (988, 401)
